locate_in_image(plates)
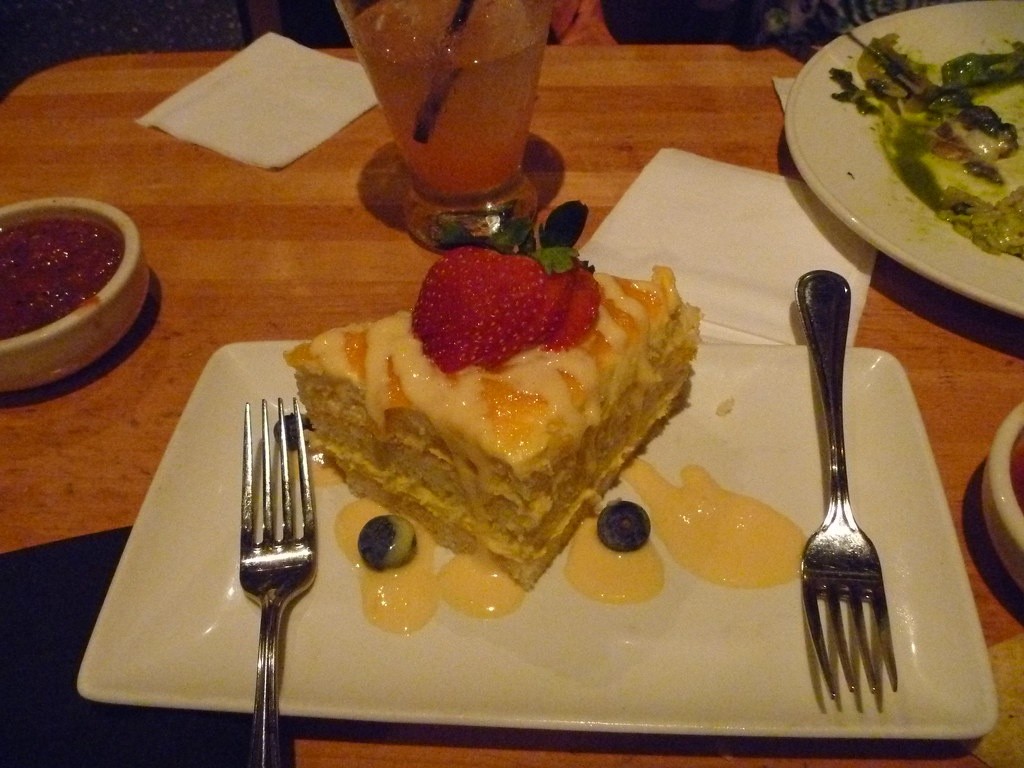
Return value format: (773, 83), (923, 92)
(784, 0), (1024, 319)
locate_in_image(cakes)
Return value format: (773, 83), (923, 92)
(287, 270), (701, 587)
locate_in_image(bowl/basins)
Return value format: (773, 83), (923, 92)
(0, 196), (151, 397)
(72, 341), (1000, 744)
(980, 403), (1024, 592)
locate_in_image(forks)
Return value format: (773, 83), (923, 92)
(792, 269), (899, 699)
(234, 395), (317, 768)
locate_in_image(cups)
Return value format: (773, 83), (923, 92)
(335, 0), (554, 251)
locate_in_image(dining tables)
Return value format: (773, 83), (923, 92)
(0, 51), (1024, 768)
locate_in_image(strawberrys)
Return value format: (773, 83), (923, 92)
(411, 245), (544, 374)
